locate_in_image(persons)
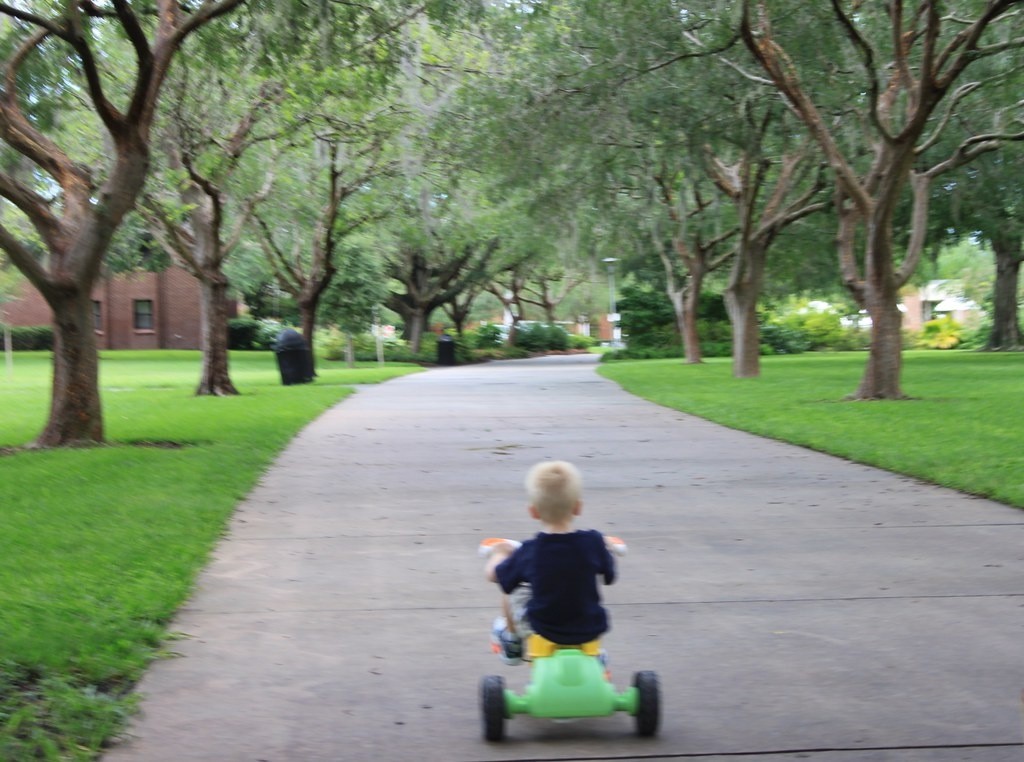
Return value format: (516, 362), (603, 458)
(487, 459), (616, 683)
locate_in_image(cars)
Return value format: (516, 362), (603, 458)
(839, 302), (908, 331)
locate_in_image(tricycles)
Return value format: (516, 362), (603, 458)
(480, 536), (665, 742)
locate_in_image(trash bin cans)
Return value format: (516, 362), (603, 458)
(436, 334), (455, 365)
(276, 328), (308, 384)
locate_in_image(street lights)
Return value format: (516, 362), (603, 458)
(601, 257), (623, 347)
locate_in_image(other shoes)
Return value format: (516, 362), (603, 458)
(491, 614), (524, 665)
(589, 650), (608, 668)
